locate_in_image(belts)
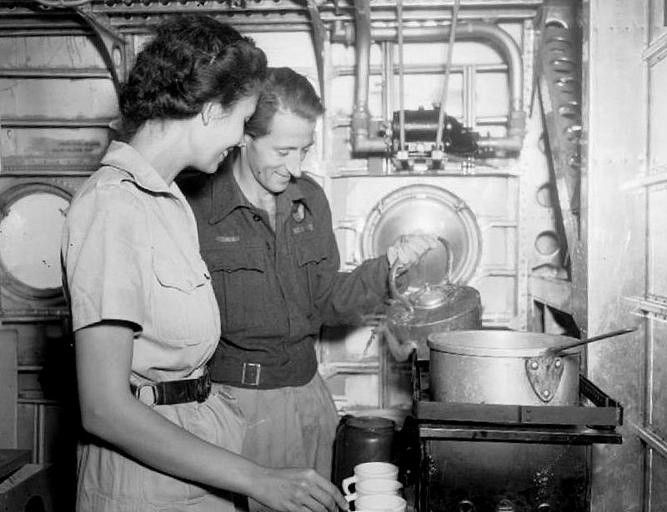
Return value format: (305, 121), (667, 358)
(209, 349), (321, 389)
(129, 372), (212, 407)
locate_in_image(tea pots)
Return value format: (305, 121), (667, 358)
(370, 233), (484, 364)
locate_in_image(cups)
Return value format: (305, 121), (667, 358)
(342, 461), (408, 512)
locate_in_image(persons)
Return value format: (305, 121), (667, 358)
(175, 67), (437, 510)
(57, 11), (353, 510)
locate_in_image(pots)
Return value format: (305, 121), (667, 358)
(426, 324), (639, 406)
